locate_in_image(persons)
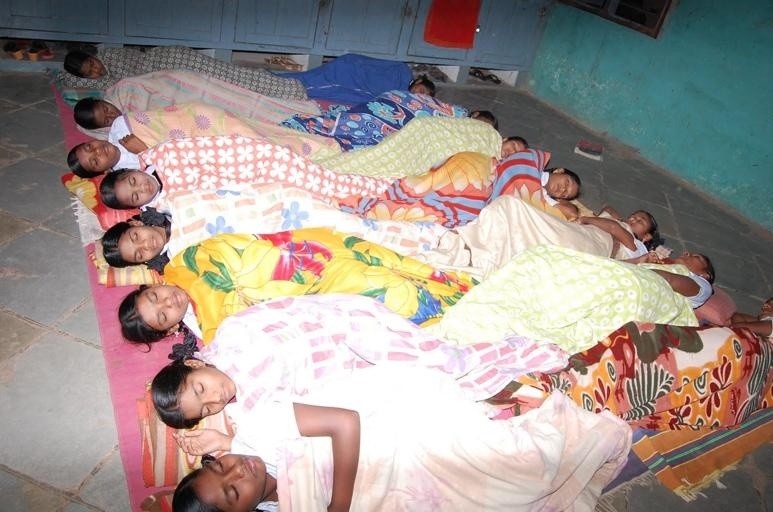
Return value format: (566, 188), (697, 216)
(171, 399), (360, 512)
(622, 246), (715, 311)
(101, 202), (191, 272)
(100, 164), (164, 213)
(542, 152), (581, 220)
(74, 96), (124, 129)
(409, 79), (435, 98)
(582, 206), (657, 257)
(118, 282), (207, 345)
(729, 296), (773, 345)
(468, 110), (498, 129)
(63, 50), (106, 80)
(501, 136), (529, 157)
(68, 116), (149, 178)
(150, 358), (238, 456)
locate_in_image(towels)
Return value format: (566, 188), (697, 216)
(424, 1), (482, 48)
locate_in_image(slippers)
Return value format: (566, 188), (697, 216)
(265, 55), (304, 71)
(27, 40), (46, 61)
(3, 42), (24, 60)
(486, 74), (501, 84)
(469, 70), (484, 81)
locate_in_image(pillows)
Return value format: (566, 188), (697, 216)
(694, 285), (737, 327)
(596, 205), (673, 261)
